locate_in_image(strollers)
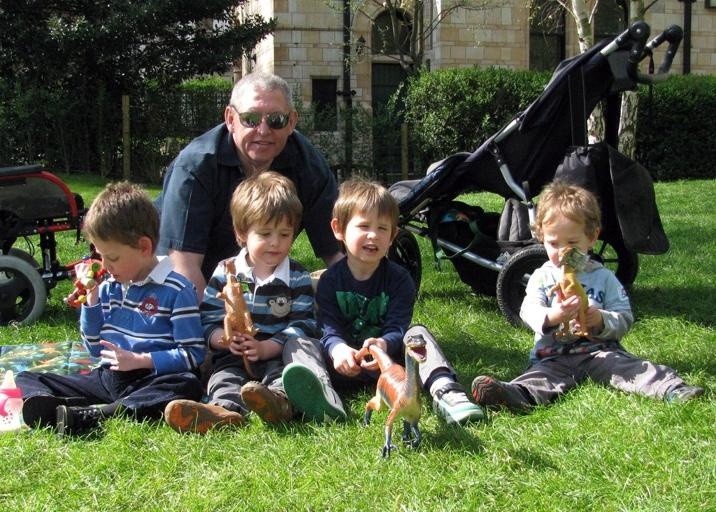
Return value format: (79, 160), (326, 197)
(387, 20), (683, 332)
(0, 164), (101, 328)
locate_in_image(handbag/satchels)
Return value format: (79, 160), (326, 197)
(554, 70), (671, 257)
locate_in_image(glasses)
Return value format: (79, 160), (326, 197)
(233, 107), (290, 129)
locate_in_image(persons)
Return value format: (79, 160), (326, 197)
(13, 177), (205, 441)
(163, 168), (319, 437)
(279, 175), (485, 427)
(150, 67), (346, 303)
(471, 177), (703, 417)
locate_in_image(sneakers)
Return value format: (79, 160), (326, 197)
(665, 383), (704, 404)
(282, 363), (347, 422)
(164, 399), (244, 435)
(55, 403), (104, 440)
(21, 394), (89, 430)
(470, 374), (533, 414)
(432, 382), (484, 424)
(241, 381), (294, 427)
